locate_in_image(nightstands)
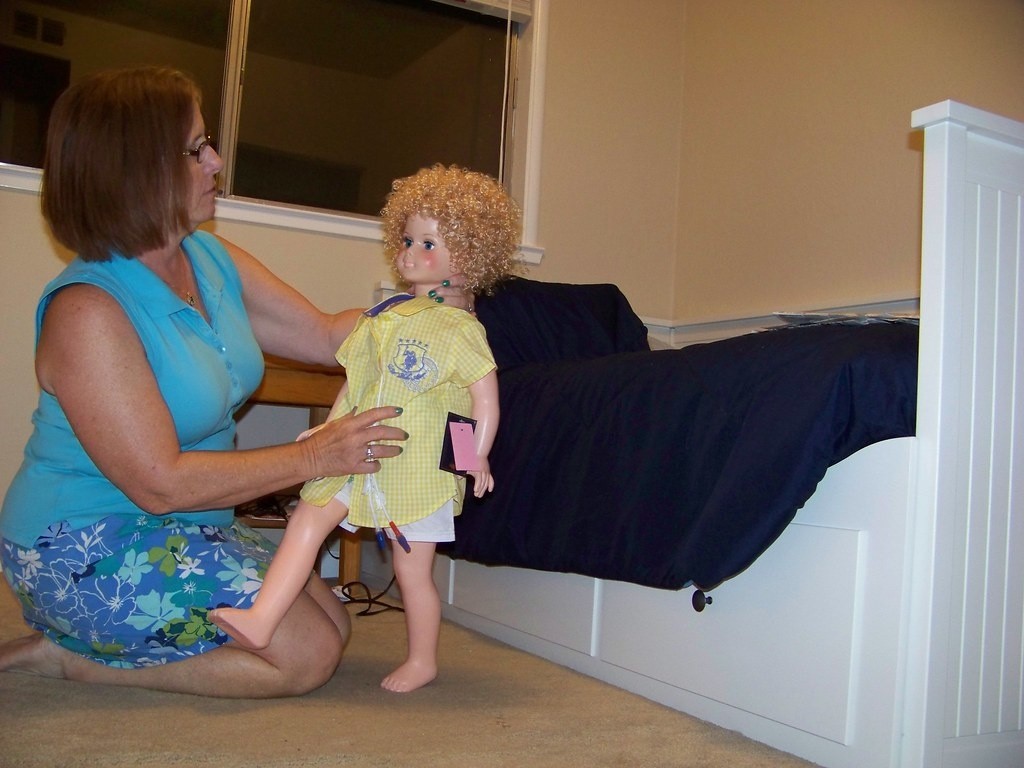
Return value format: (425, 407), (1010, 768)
(239, 354), (361, 597)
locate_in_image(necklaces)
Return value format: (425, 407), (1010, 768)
(166, 244), (196, 306)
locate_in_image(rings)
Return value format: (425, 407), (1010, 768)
(469, 303), (472, 312)
(365, 444), (374, 462)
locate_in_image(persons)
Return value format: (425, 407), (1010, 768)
(0, 67), (478, 698)
(206, 165), (518, 693)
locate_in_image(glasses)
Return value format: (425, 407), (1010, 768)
(181, 130), (213, 164)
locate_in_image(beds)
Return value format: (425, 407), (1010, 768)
(360, 98), (1024, 768)
